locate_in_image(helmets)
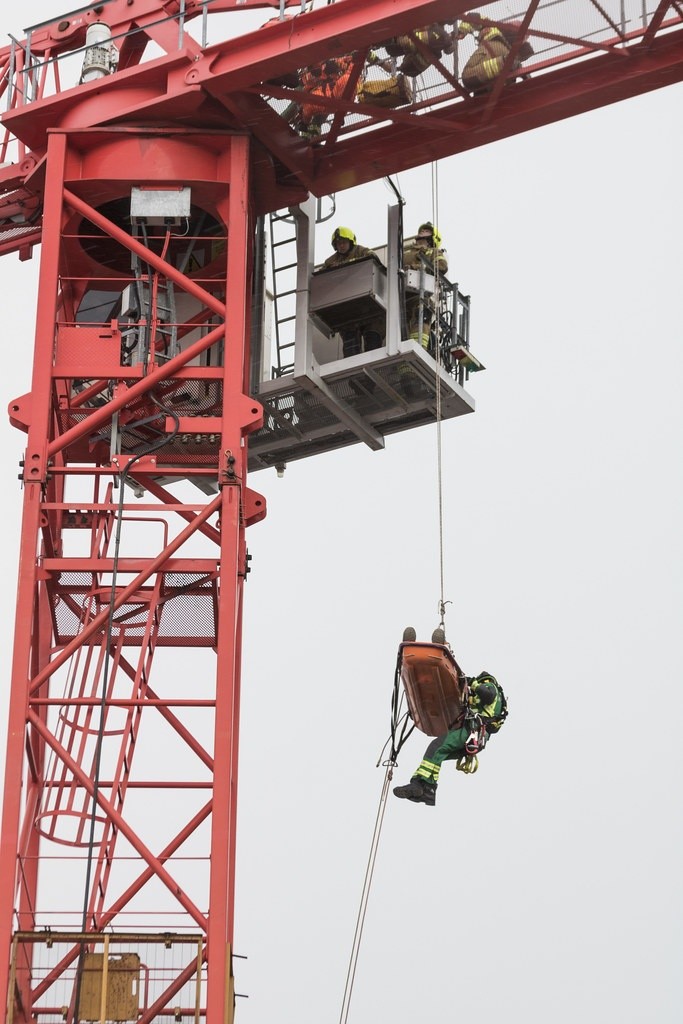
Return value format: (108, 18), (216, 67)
(418, 222), (441, 247)
(331, 226), (357, 251)
(478, 671), (496, 685)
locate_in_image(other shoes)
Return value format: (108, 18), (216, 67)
(513, 60), (532, 83)
(408, 783), (437, 806)
(300, 127), (321, 140)
(393, 778), (423, 798)
(444, 43), (454, 55)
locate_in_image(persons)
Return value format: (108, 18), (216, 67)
(392, 670), (509, 806)
(322, 228), (383, 360)
(403, 221), (447, 347)
(260, 13), (535, 146)
